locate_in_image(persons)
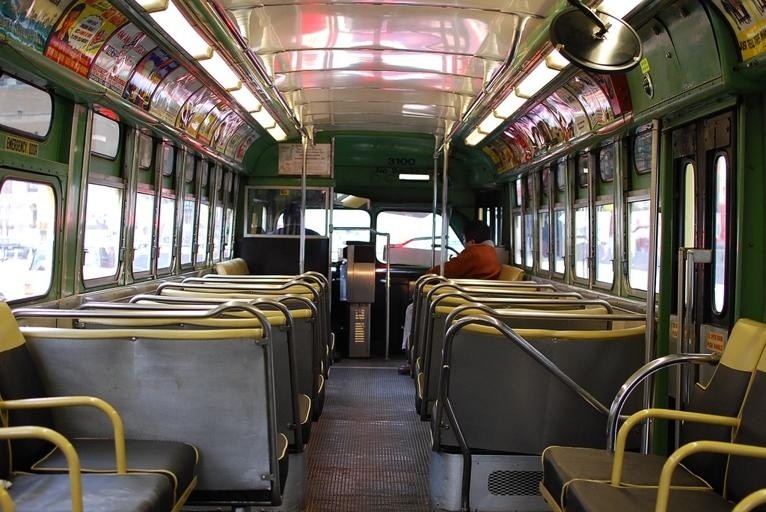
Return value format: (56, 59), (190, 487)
(262, 203), (319, 235)
(399, 221), (502, 373)
(54, 2), (86, 42)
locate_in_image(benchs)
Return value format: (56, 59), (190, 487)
(216, 257), (249, 274)
(501, 262), (525, 280)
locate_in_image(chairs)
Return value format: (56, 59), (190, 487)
(0, 303), (198, 510)
(538, 318), (765, 512)
(563, 346), (766, 512)
(1, 397), (173, 511)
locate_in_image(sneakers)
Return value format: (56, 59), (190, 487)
(398, 360), (411, 375)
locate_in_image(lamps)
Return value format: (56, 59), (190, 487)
(465, 0), (648, 146)
(135, 0), (289, 143)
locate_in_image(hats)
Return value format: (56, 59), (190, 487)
(284, 204), (299, 215)
